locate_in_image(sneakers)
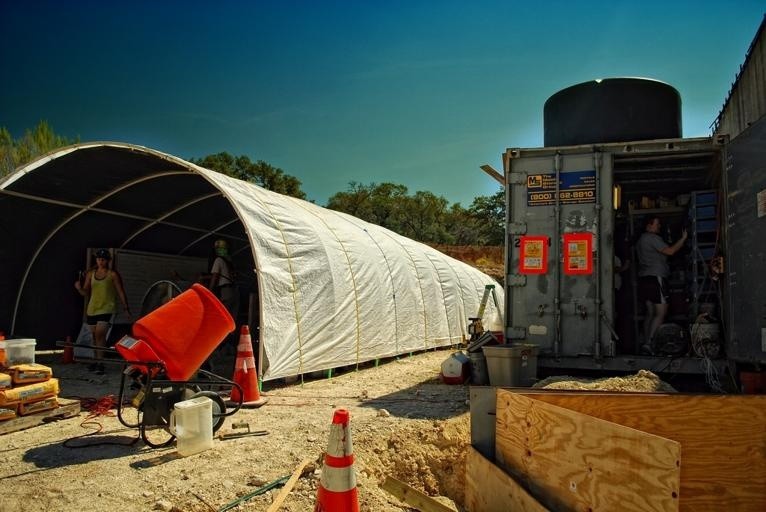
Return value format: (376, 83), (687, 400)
(84, 361), (105, 374)
(642, 342), (656, 355)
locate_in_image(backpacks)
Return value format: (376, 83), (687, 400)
(217, 256), (240, 282)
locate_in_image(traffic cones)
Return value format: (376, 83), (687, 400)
(221, 324), (270, 410)
(312, 407), (361, 512)
(60, 336), (77, 364)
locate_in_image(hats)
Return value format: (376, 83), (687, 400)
(94, 249), (111, 260)
(214, 239), (230, 249)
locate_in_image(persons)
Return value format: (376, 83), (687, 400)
(73, 248), (132, 375)
(208, 239), (241, 321)
(615, 213), (688, 357)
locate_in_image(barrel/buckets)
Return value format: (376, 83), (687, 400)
(0, 336), (6, 366)
(6, 338), (36, 364)
(740, 370), (766, 394)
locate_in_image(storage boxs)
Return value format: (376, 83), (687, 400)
(0, 338), (37, 364)
(481, 342), (540, 387)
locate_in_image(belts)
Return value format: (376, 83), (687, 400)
(220, 283), (232, 288)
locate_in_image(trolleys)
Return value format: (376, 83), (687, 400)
(53, 338), (245, 450)
